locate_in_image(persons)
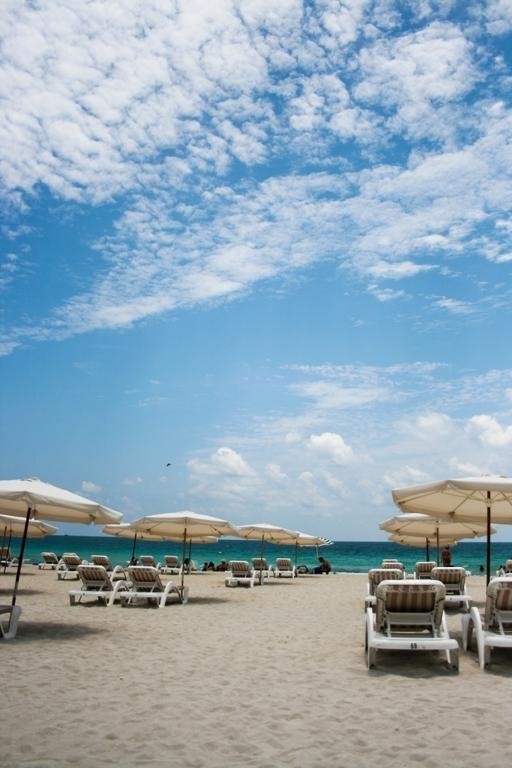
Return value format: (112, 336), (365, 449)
(441, 545), (452, 567)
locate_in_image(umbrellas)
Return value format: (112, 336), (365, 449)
(0, 477), (123, 605)
(102, 510), (335, 599)
(392, 472), (512, 586)
(378, 513), (496, 568)
(388, 534), (458, 562)
(0, 513), (58, 574)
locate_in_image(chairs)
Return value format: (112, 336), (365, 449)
(38, 547), (189, 609)
(364, 559), (512, 671)
(0, 604), (22, 639)
(0, 547), (30, 570)
(224, 558), (296, 588)
